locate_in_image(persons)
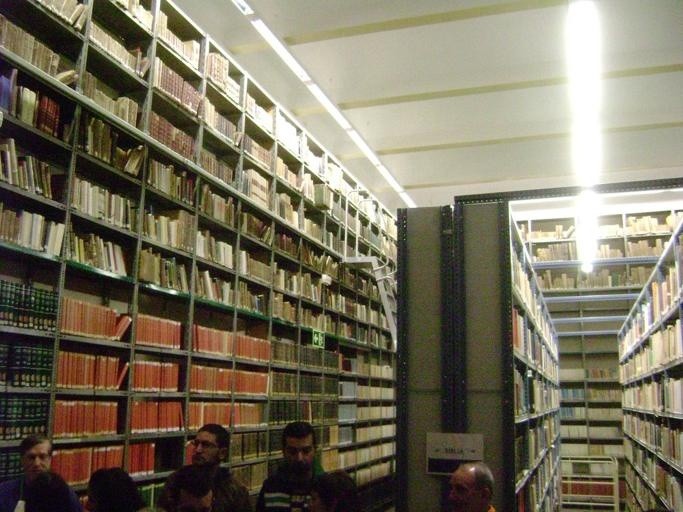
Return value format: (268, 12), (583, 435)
(432, 463), (500, 512)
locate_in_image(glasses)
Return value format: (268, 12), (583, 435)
(191, 440), (219, 449)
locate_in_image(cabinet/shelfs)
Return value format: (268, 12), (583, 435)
(1, 0), (396, 490)
(397, 187), (683, 510)
